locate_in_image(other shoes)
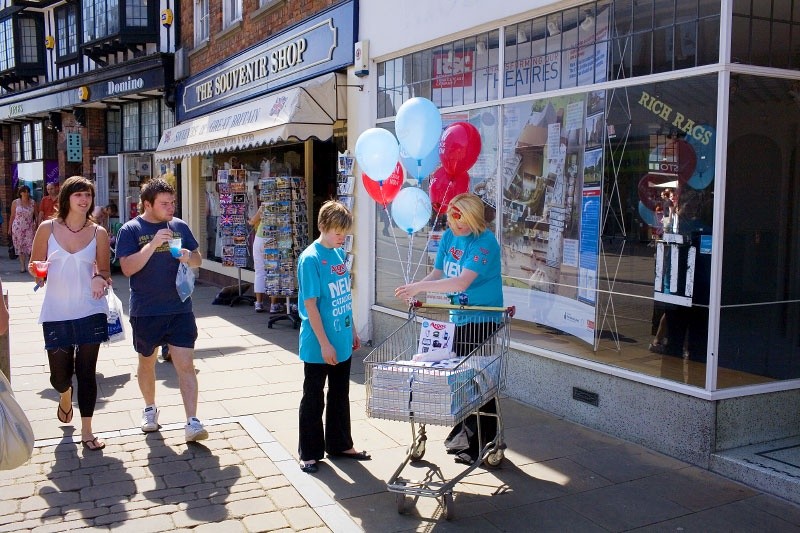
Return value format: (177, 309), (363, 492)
(164, 350), (171, 360)
(454, 454), (475, 464)
(330, 448), (372, 460)
(299, 459), (318, 472)
(649, 343), (665, 353)
(20, 267), (26, 272)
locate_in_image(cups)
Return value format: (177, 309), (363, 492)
(168, 238), (182, 258)
(32, 261), (50, 277)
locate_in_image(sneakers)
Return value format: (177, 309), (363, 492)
(270, 303), (284, 312)
(184, 418), (209, 441)
(254, 301), (265, 311)
(140, 406), (160, 431)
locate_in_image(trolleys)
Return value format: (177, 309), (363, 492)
(363, 300), (517, 521)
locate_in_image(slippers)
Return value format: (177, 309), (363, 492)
(59, 386), (74, 423)
(82, 438), (102, 450)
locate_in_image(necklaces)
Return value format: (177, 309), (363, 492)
(62, 216), (87, 233)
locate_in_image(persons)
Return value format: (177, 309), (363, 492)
(8, 176), (209, 450)
(528, 206), (566, 334)
(250, 205), (284, 313)
(647, 186), (698, 354)
(295, 202), (372, 471)
(394, 195), (504, 464)
(379, 203), (397, 237)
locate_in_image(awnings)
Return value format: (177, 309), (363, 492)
(152, 72), (349, 165)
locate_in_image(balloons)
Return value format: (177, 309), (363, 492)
(638, 125), (716, 228)
(357, 97), (482, 233)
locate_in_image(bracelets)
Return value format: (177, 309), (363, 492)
(92, 274), (106, 281)
(188, 250), (191, 259)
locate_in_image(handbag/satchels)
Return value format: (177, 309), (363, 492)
(175, 260), (195, 302)
(102, 285), (126, 345)
(7, 235), (19, 260)
(0, 369), (34, 470)
